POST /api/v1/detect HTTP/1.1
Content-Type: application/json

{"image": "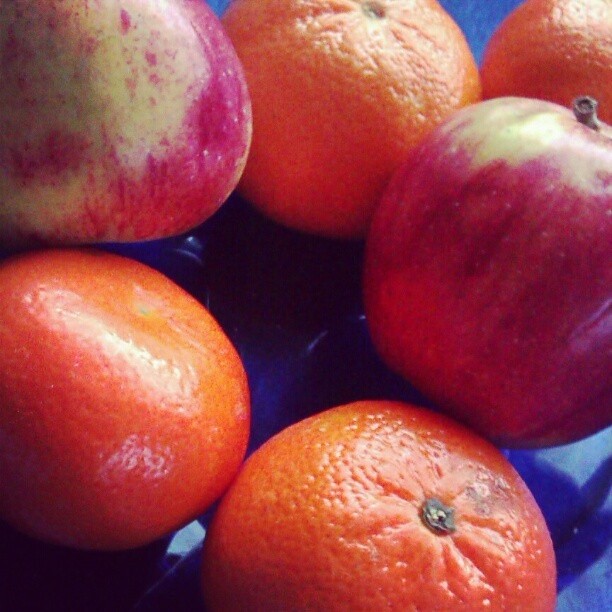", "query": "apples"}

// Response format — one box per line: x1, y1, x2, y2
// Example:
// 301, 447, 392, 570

363, 95, 612, 450
0, 0, 252, 246
